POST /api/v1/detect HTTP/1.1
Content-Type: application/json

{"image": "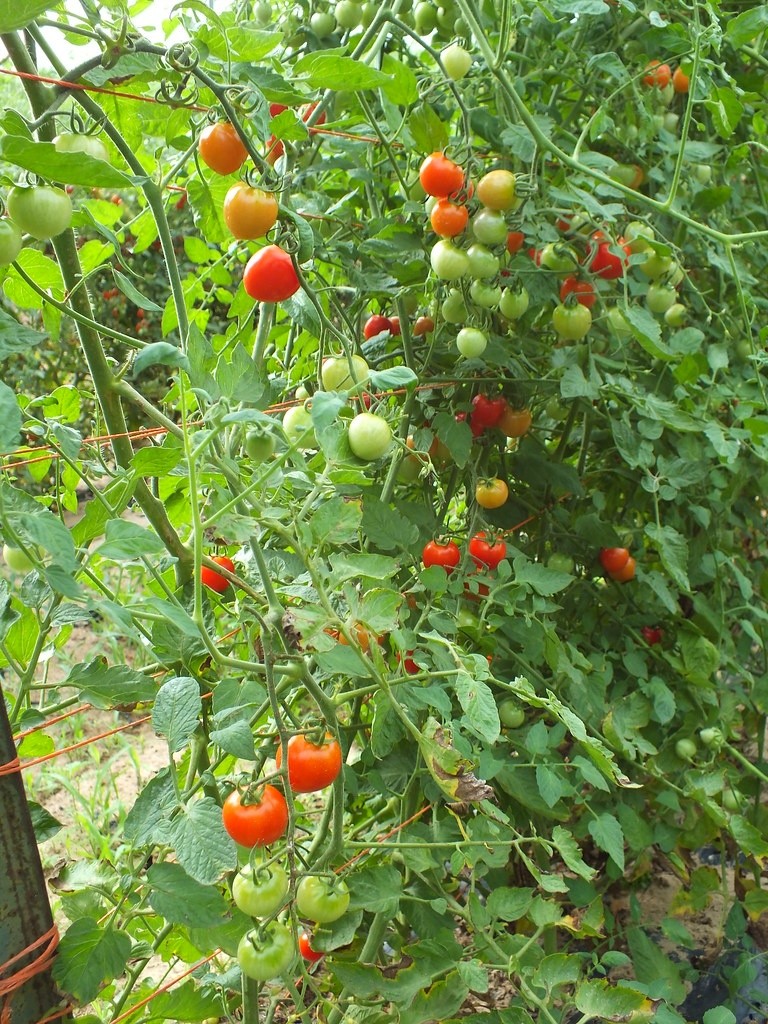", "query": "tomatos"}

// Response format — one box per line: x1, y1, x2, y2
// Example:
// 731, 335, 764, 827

1, 0, 766, 1024
